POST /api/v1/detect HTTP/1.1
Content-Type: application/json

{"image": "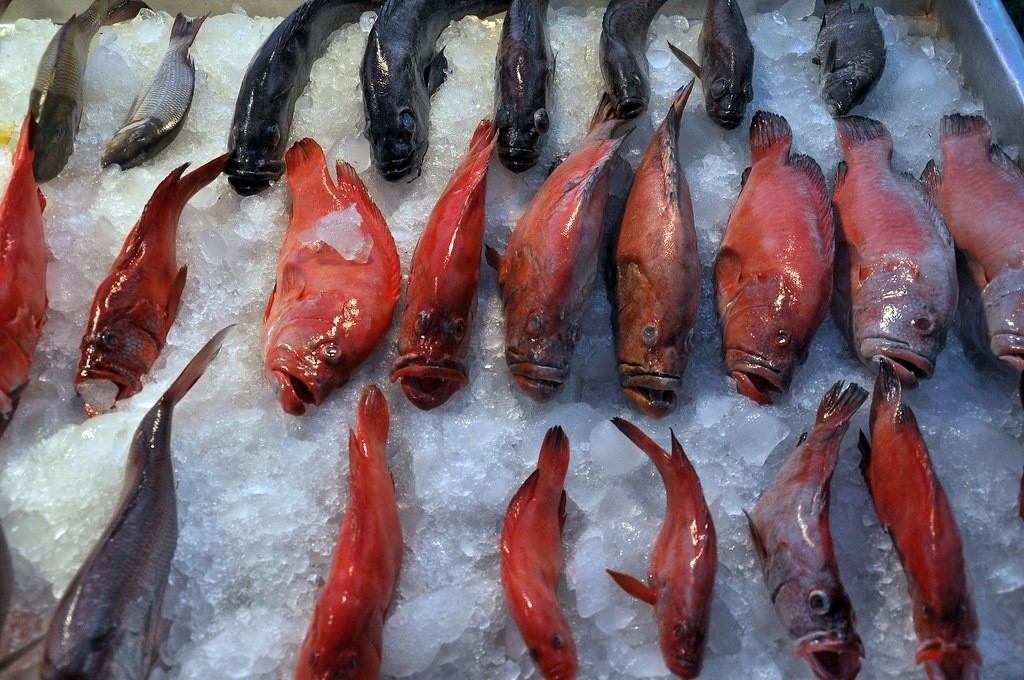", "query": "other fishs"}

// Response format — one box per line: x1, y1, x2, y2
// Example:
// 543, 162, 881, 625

0, 1, 1023, 680
